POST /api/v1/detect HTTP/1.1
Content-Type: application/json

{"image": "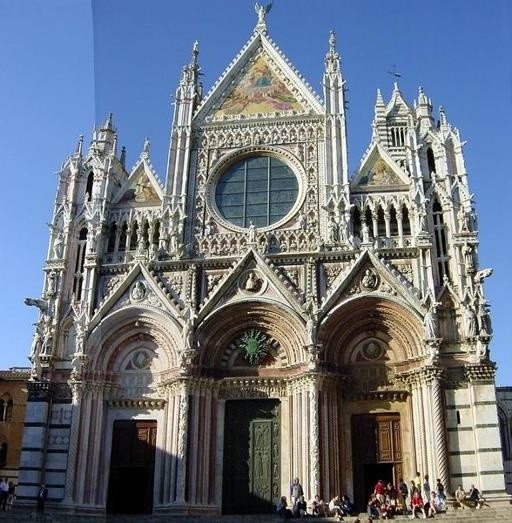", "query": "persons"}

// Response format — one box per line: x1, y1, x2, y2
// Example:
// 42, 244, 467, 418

205, 216, 215, 235
276, 472, 482, 523
477, 304, 494, 336
0, 476, 9, 512
132, 282, 144, 300
294, 210, 307, 229
246, 273, 258, 289
248, 220, 257, 243
361, 269, 375, 287
253, 65, 272, 86
463, 303, 477, 337
5, 480, 15, 512
36, 484, 49, 515
